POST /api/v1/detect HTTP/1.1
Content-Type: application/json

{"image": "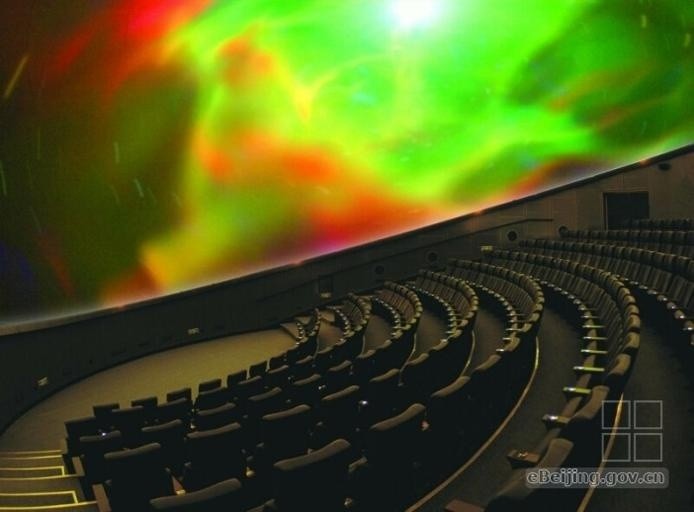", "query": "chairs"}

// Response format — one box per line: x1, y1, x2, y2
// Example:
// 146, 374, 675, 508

62, 213, 692, 511
